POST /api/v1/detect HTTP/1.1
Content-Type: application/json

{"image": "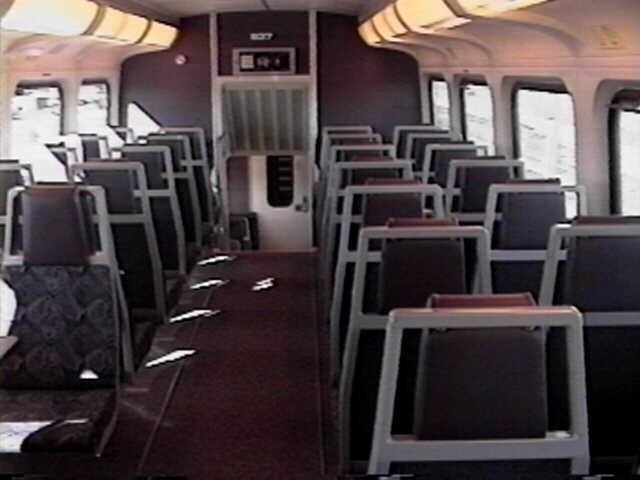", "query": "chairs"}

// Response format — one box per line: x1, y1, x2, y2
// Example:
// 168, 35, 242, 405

318, 120, 640, 479
0, 125, 230, 470
